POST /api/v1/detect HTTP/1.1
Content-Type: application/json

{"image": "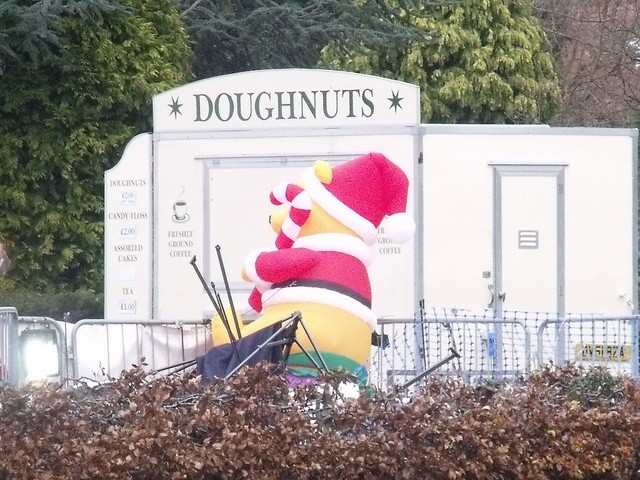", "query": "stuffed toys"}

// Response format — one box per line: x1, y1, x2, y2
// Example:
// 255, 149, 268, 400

210, 152, 409, 397
